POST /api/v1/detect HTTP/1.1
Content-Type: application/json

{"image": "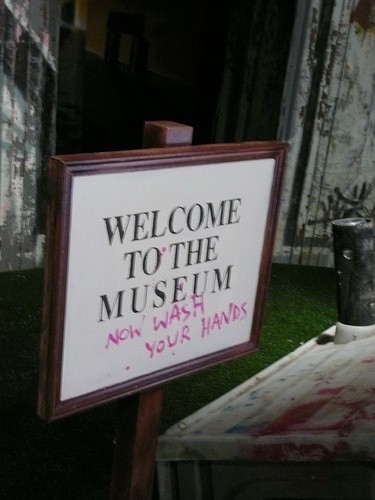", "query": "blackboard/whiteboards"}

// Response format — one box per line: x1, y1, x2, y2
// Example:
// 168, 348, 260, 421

34, 140, 290, 427
